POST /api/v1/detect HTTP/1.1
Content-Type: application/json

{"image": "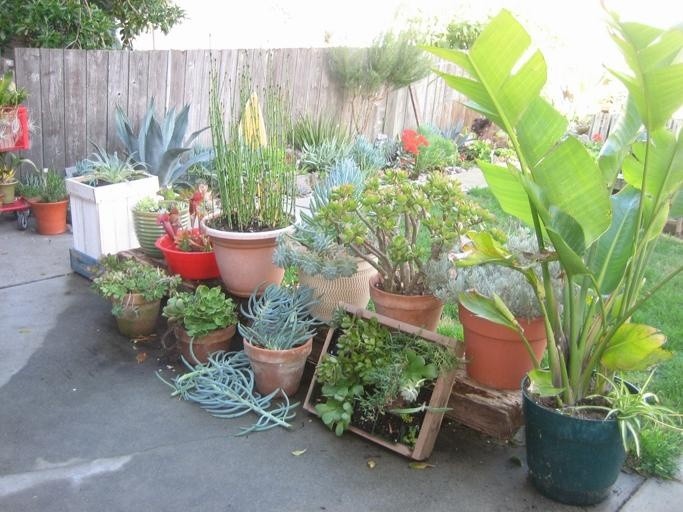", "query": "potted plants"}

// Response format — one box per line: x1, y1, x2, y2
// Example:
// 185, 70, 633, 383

197, 45, 299, 298
423, 1, 681, 504
323, 168, 480, 333
0, 167, 19, 205
237, 287, 318, 396
156, 190, 218, 282
162, 283, 236, 366
306, 303, 459, 463
274, 162, 384, 323
29, 168, 68, 235
456, 233, 566, 392
0, 70, 30, 153
133, 189, 190, 257
95, 253, 178, 339
67, 138, 161, 280
